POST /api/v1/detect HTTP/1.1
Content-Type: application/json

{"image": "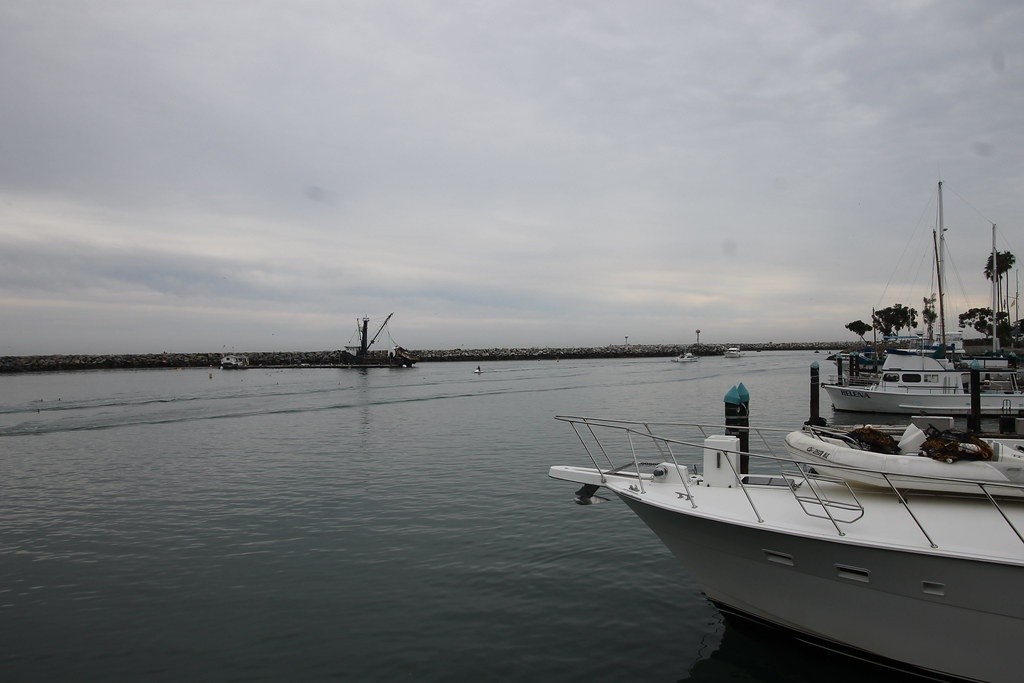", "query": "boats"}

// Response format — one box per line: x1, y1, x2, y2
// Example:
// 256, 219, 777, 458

548, 415, 1024, 682
340, 312, 416, 364
474, 370, 484, 372
725, 347, 740, 358
821, 179, 1024, 417
671, 346, 698, 362
